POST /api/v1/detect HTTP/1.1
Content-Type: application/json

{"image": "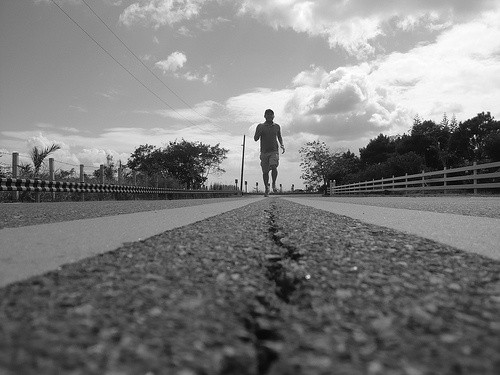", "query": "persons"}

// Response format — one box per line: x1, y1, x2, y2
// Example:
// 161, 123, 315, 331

254, 108, 285, 198
